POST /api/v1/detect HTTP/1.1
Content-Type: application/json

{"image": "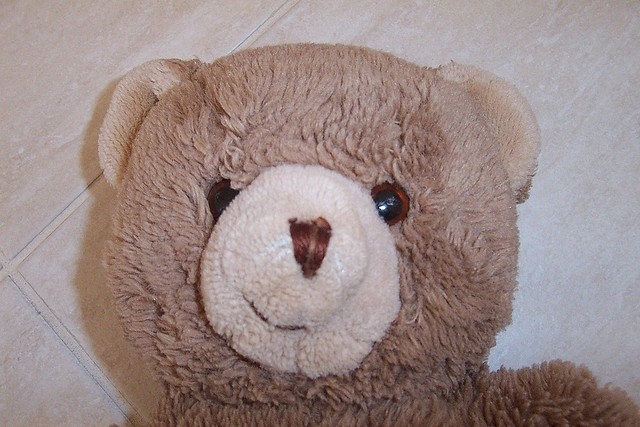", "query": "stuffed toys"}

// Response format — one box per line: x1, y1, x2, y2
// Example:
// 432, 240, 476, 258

96, 42, 639, 427
